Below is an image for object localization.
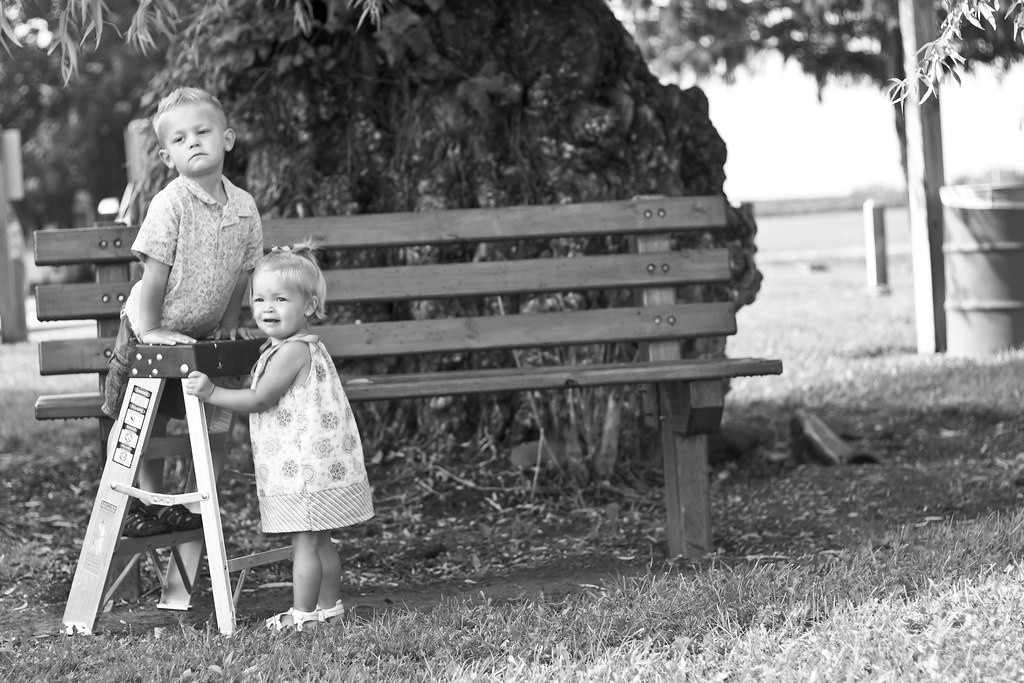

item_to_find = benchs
[35,195,784,607]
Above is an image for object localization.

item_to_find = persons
[185,243,377,631]
[97,86,265,537]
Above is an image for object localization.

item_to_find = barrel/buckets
[940,180,1022,356]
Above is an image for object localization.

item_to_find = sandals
[122,502,171,536]
[317,600,350,626]
[266,606,320,634]
[154,504,204,530]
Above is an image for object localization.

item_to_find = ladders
[60,346,241,639]
